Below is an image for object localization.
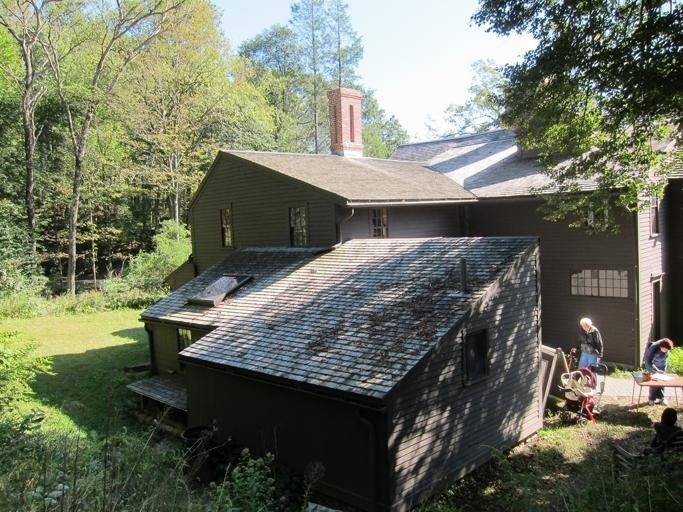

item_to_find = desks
[630,372,683,417]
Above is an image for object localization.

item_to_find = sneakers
[649,398,668,406]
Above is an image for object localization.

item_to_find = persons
[644,336,675,406]
[571,317,604,389]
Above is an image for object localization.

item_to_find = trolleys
[561,360,610,428]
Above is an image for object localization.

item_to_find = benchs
[609,441,683,486]
